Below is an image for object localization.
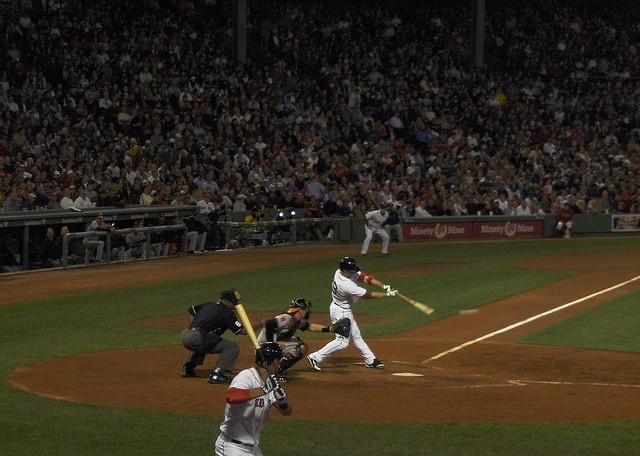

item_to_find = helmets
[286,298,312,313]
[340,256,361,272]
[256,342,283,360]
[221,289,240,305]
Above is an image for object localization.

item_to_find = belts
[230,439,255,446]
[334,301,351,309]
[189,327,210,332]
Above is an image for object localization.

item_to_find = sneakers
[181,366,196,376]
[278,375,288,383]
[307,355,322,371]
[207,372,232,383]
[366,358,385,369]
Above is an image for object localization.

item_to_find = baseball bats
[234,303,272,375]
[396,292,434,315]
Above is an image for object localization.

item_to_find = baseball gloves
[331,318,350,338]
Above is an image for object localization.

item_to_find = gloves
[386,289,398,297]
[383,285,391,290]
[273,386,287,405]
[261,374,279,394]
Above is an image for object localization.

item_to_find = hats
[381,202,387,208]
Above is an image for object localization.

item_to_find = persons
[168,216,186,255]
[180,289,266,384]
[384,205,403,243]
[576,200,640,216]
[360,201,390,256]
[57,226,72,256]
[555,0,640,201]
[125,221,150,258]
[154,213,171,257]
[271,209,287,245]
[240,208,268,248]
[306,256,398,370]
[215,342,293,456]
[41,228,60,262]
[556,202,575,238]
[84,212,116,263]
[186,214,208,255]
[254,297,350,383]
[303,195,323,238]
[286,207,298,241]
[323,192,350,240]
